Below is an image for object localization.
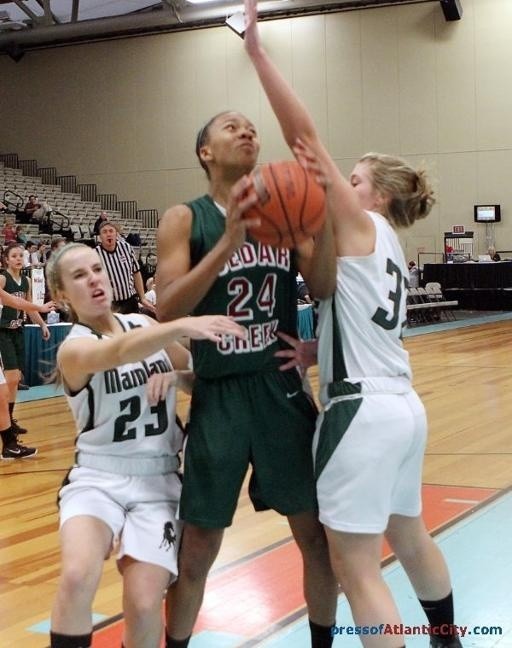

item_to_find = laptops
[479,255,493,262]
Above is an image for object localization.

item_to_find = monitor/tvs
[474,204,500,223]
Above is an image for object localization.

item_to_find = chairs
[0,162,157,255]
[404,284,458,326]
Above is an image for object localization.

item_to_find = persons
[46,240,247,648]
[231,0,463,648]
[486,246,501,262]
[155,111,340,647]
[296,280,312,304]
[408,260,419,288]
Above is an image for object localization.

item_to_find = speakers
[440,0,463,21]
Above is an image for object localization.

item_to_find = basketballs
[242,159,327,248]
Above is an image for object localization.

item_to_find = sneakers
[2,446,38,459]
[12,420,27,434]
